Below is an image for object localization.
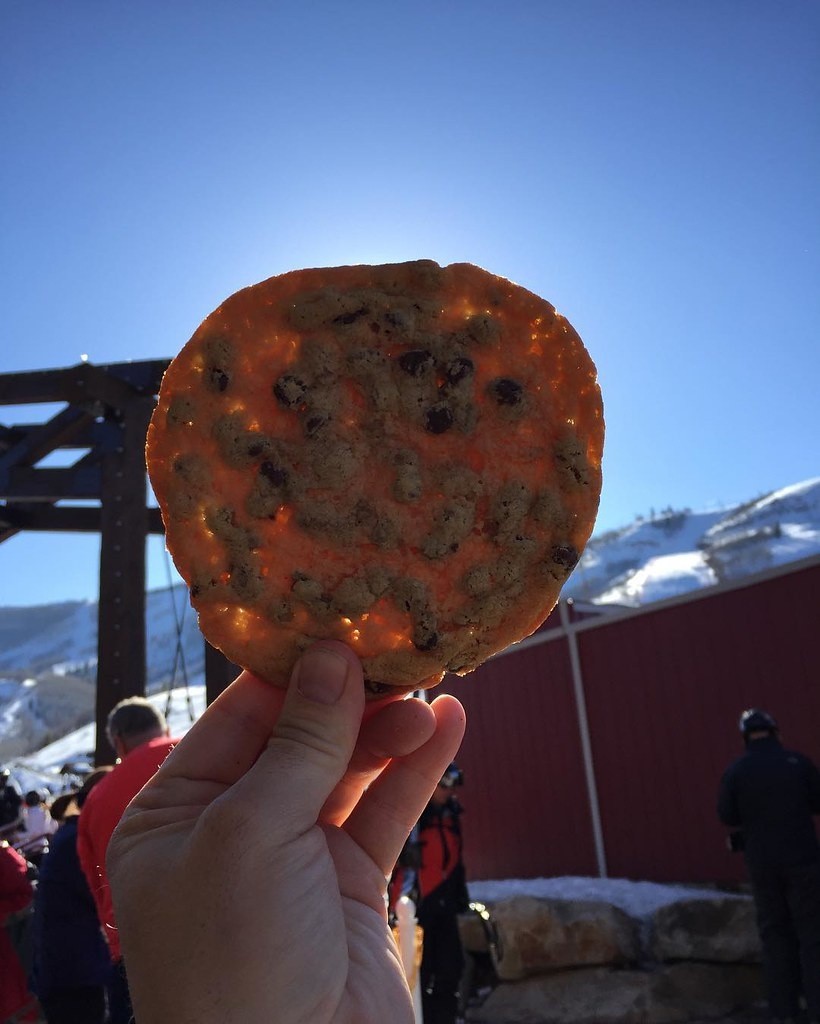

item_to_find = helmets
[738,708,777,739]
[439,764,466,790]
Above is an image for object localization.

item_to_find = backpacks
[455,903,504,1005]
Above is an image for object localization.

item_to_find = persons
[719,709,820,1016]
[76,697,191,973]
[102,655,467,1024]
[386,755,475,1024]
[0,697,139,1024]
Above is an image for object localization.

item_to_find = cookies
[145,259,607,699]
